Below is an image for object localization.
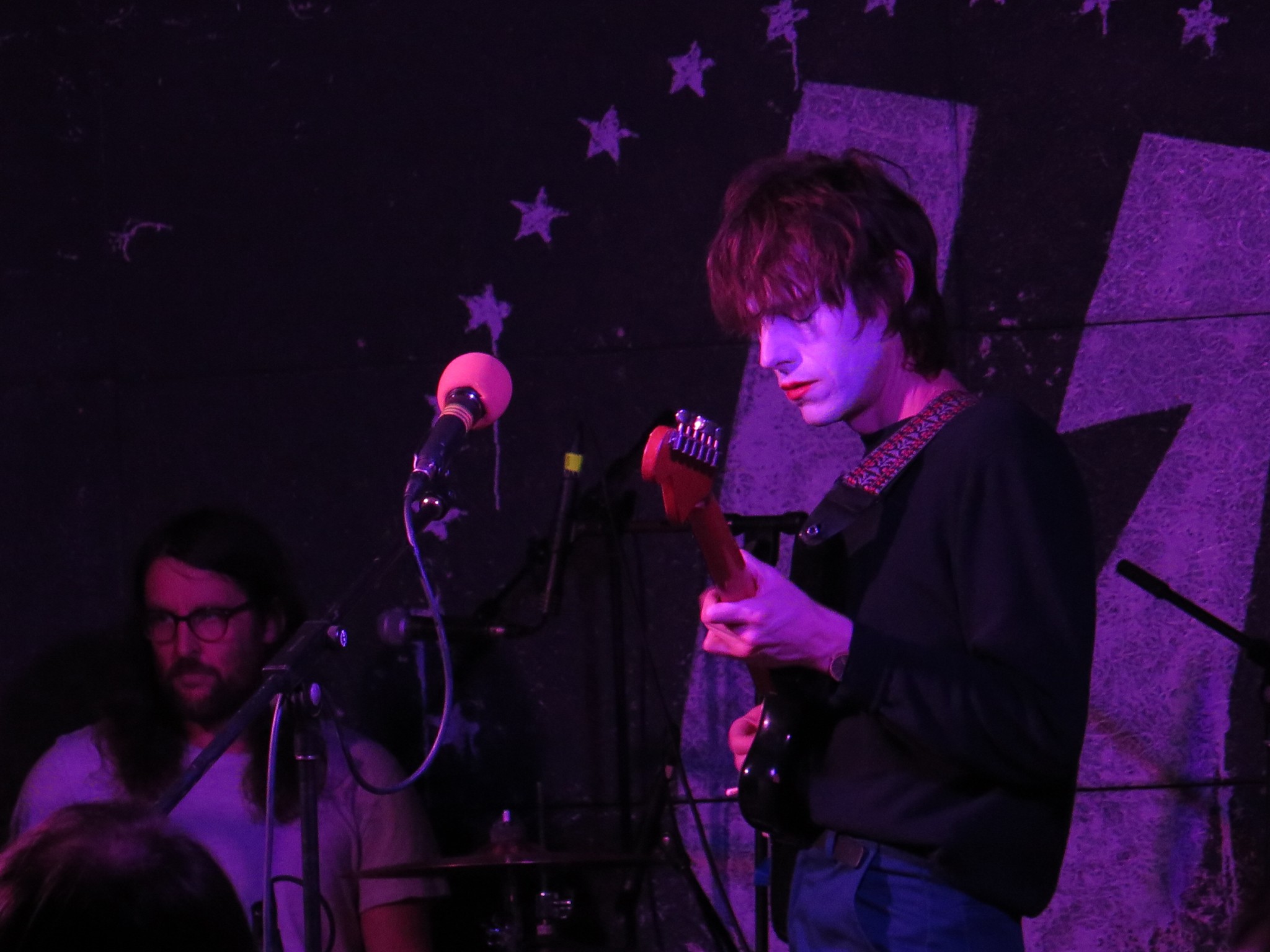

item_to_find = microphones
[374,609,510,645]
[405,352,514,497]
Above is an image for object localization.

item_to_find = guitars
[640,410,833,944]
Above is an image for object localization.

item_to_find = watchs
[825,651,851,683]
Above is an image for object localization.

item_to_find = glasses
[134,599,256,642]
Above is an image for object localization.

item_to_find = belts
[784,824,950,884]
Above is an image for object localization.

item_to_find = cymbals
[357,843,664,879]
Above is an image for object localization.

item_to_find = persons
[696,149,1095,952]
[0,509,452,952]
[0,799,265,952]
